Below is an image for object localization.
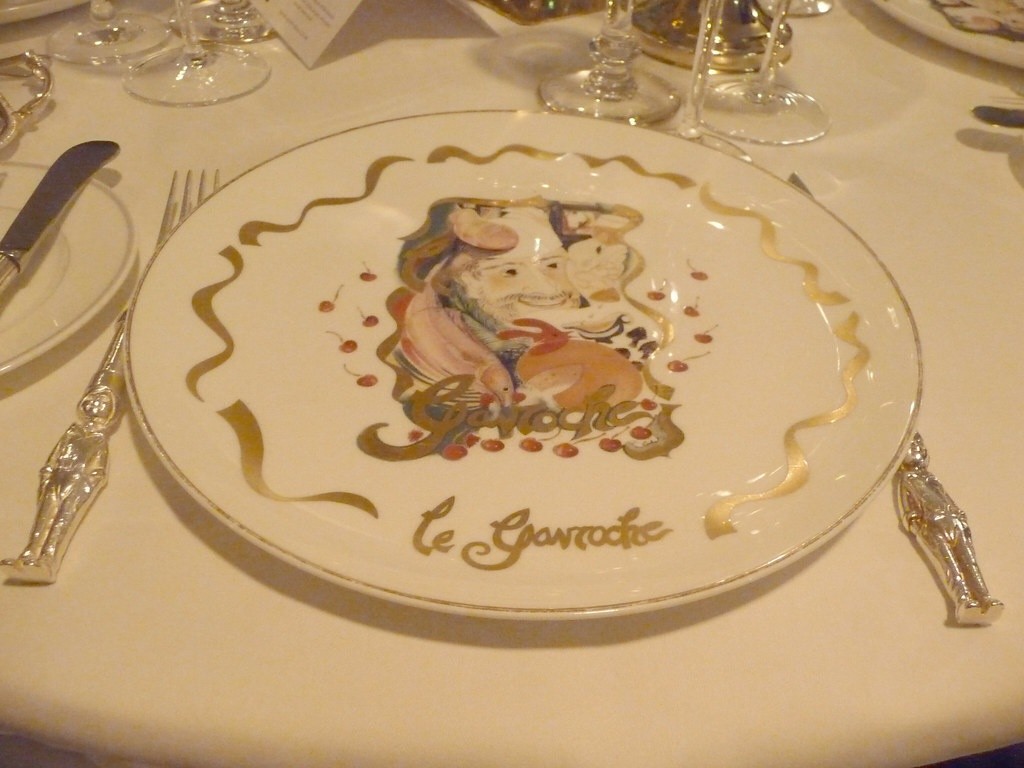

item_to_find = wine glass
[44,1,173,67]
[123,0,273,106]
[169,0,274,44]
[658,0,752,162]
[537,0,682,124]
[696,0,832,145]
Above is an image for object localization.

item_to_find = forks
[1,169,223,583]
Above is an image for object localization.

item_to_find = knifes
[788,173,1002,626]
[0,141,120,292]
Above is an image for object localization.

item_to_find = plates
[0,164,140,376]
[122,110,924,620]
[871,0,1024,70]
